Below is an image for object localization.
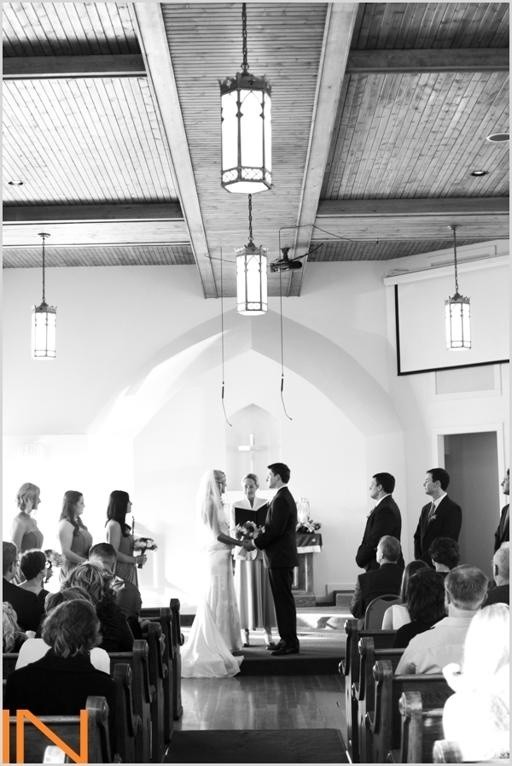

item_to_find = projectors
[271,250,304,274]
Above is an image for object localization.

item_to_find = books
[234,501,269,529]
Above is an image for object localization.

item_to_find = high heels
[264,632,274,646]
[243,632,249,646]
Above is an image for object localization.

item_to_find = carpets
[163,726,349,763]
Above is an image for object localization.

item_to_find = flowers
[133,536,159,569]
[233,521,258,560]
[44,549,64,583]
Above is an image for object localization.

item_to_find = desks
[292,531,322,595]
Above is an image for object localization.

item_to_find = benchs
[337,615,458,762]
[4,595,199,762]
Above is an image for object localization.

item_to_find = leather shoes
[266,639,283,650]
[272,642,299,655]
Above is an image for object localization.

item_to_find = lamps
[234,192,270,316]
[31,234,57,360]
[444,223,475,350]
[217,2,274,195]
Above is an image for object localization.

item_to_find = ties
[427,504,435,521]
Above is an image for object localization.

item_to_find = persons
[4,539,166,764]
[493,469,509,551]
[13,484,44,559]
[105,491,146,589]
[201,468,247,657]
[414,466,463,563]
[231,472,276,651]
[343,532,511,762]
[242,462,300,655]
[355,472,405,575]
[58,491,93,574]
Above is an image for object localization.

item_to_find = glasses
[44,562,50,569]
